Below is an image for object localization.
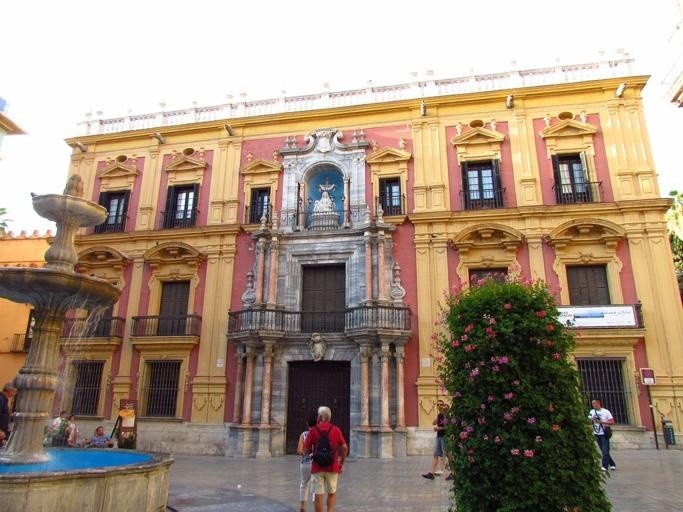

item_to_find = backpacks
[312,423,337,469]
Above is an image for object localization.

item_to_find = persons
[296,413,315,512]
[421,399,455,480]
[433,404,452,476]
[52,410,66,429]
[312,191,336,214]
[587,399,616,470]
[303,406,348,512]
[66,415,79,446]
[89,425,113,447]
[0,382,16,447]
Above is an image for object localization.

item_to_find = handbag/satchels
[603,427,613,440]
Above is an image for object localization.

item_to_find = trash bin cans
[662,419,675,445]
[51,434,65,447]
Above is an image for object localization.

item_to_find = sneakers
[601,463,617,472]
[420,469,456,481]
